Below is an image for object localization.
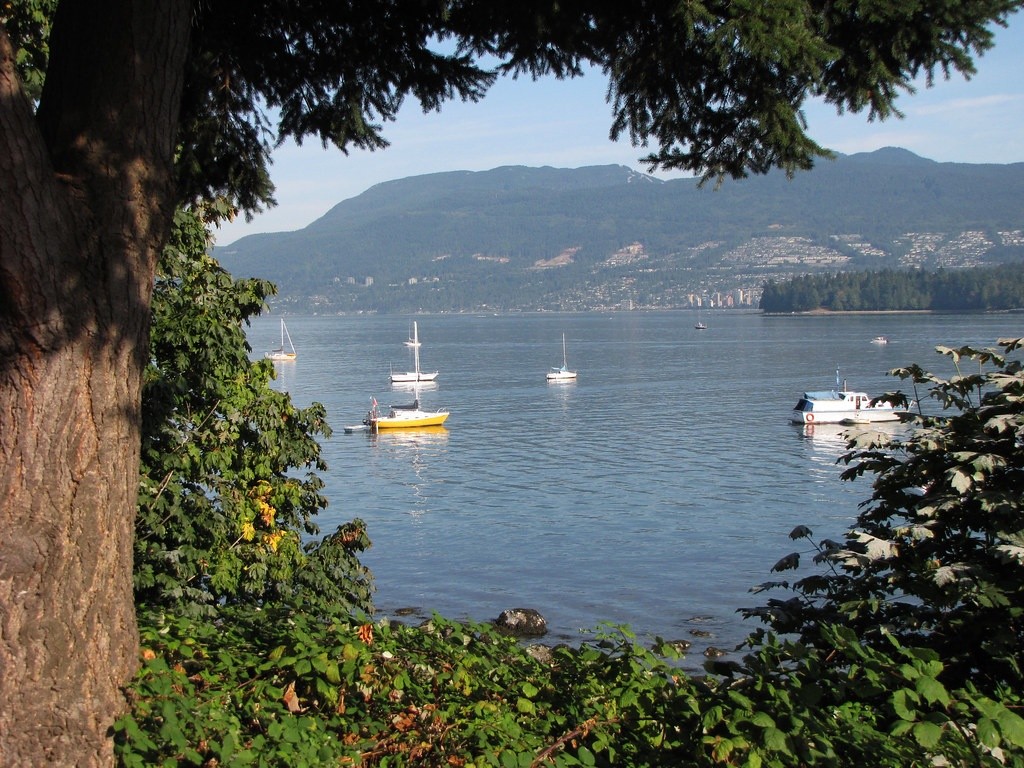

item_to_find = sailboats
[263,316,299,361]
[401,318,422,347]
[544,332,579,380]
[387,321,440,382]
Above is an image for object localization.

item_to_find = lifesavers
[806,424,814,430]
[807,414,814,422]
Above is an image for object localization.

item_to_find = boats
[343,423,370,433]
[870,336,889,345]
[785,366,917,426]
[694,321,708,330]
[362,372,449,429]
